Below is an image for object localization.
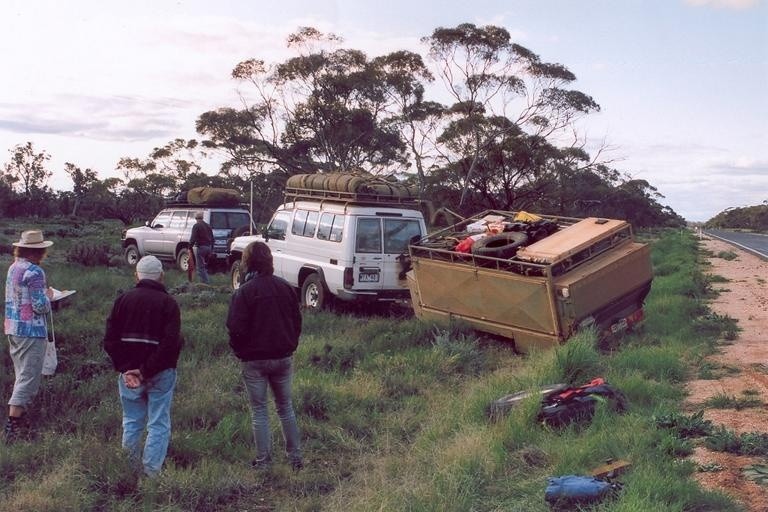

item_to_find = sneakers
[250,455,274,467]
[290,457,304,470]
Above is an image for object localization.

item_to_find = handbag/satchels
[40,340,59,376]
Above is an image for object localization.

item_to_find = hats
[11,229,55,250]
[135,255,164,282]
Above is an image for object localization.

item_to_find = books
[49,286,76,302]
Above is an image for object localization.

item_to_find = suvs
[226,187,431,315]
[118,196,262,276]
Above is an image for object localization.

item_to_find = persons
[3,229,54,434]
[104,255,181,476]
[188,213,214,284]
[226,241,302,465]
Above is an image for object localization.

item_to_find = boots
[4,416,39,442]
